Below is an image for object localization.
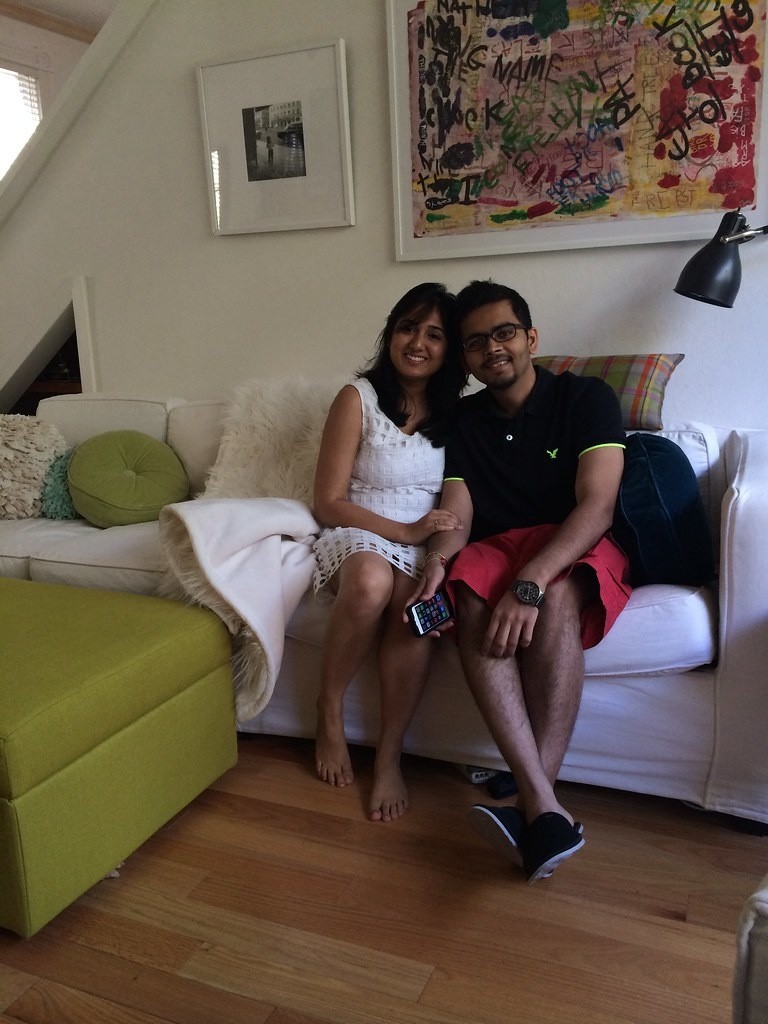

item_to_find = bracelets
[423,550,448,568]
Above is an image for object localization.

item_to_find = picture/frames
[386,0,768,263]
[195,38,356,238]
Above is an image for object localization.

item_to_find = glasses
[462,323,525,352]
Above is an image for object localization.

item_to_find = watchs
[509,579,545,609]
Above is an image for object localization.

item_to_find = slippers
[470,804,524,867]
[522,812,584,888]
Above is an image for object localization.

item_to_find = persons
[265,135,274,162]
[401,277,633,883]
[290,282,460,821]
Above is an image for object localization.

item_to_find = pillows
[43,445,78,521]
[0,413,69,521]
[67,427,190,529]
[530,353,686,431]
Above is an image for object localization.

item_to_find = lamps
[674,201,768,309]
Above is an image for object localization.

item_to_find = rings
[435,519,438,525]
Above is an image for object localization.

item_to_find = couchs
[0,392,767,827]
[0,576,242,938]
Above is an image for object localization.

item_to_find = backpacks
[611,432,713,587]
